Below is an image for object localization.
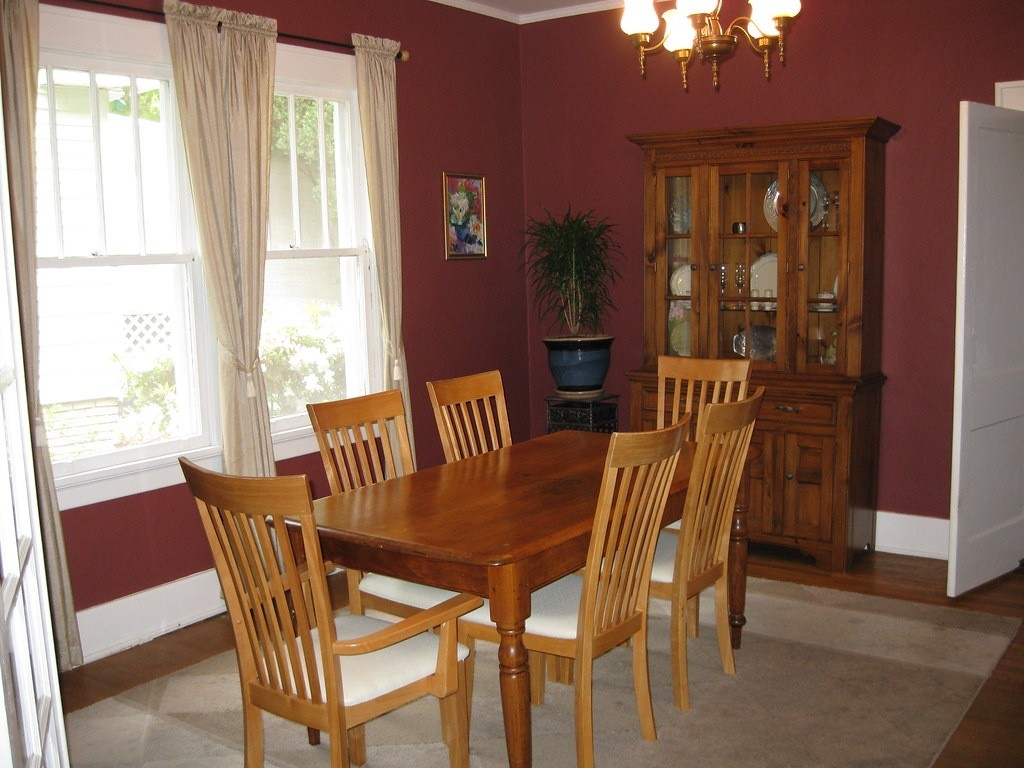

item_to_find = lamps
[620,1,804,92]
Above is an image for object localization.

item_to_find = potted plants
[521,206,623,397]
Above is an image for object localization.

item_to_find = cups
[732,222,746,233]
[749,288,759,310]
[816,290,834,311]
[764,289,774,310]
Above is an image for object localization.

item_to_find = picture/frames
[442,171,490,261]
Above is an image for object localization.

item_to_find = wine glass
[732,262,746,310]
[718,262,729,310]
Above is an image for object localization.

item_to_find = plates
[672,321,691,357]
[750,252,778,309]
[733,326,776,360]
[670,265,690,311]
[763,172,828,234]
[669,192,690,233]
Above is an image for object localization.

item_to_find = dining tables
[262,417,762,768]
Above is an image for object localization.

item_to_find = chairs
[453,408,695,768]
[175,452,474,766]
[305,387,482,741]
[636,354,754,641]
[428,368,515,461]
[581,381,768,713]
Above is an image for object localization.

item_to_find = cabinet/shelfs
[629,113,901,577]
